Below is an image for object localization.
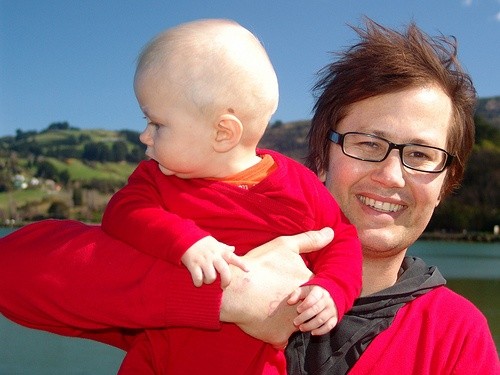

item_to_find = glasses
[328,128,458,174]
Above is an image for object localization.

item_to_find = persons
[101,17,363,375]
[0,13,500,375]
